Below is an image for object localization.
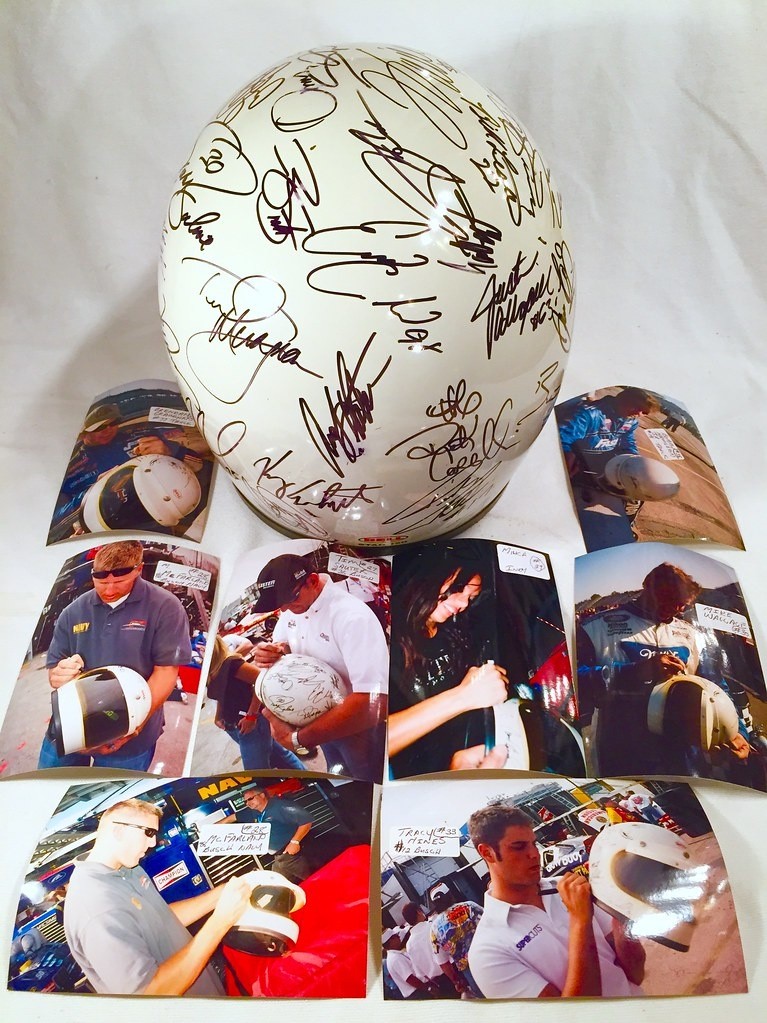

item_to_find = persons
[574,561,751,778]
[37,538,195,772]
[387,547,513,783]
[576,603,620,626]
[50,402,212,543]
[59,798,252,999]
[212,786,318,887]
[203,554,390,784]
[382,789,664,1000]
[556,386,687,500]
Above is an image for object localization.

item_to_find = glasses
[91,418,121,432]
[111,821,159,839]
[244,792,262,803]
[286,576,307,605]
[91,560,143,579]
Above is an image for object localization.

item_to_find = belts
[223,724,240,732]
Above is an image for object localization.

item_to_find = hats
[382,925,402,944]
[430,883,450,901]
[251,553,316,615]
[81,403,125,432]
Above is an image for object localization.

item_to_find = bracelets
[247,712,260,717]
[245,715,258,721]
[427,986,431,991]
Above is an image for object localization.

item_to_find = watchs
[291,731,310,755]
[290,840,300,845]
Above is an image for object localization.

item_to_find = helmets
[646,674,738,751]
[255,653,347,729]
[154,44,576,549]
[465,697,586,777]
[604,453,680,502]
[584,823,712,952]
[49,664,152,759]
[219,868,305,957]
[78,453,201,533]
